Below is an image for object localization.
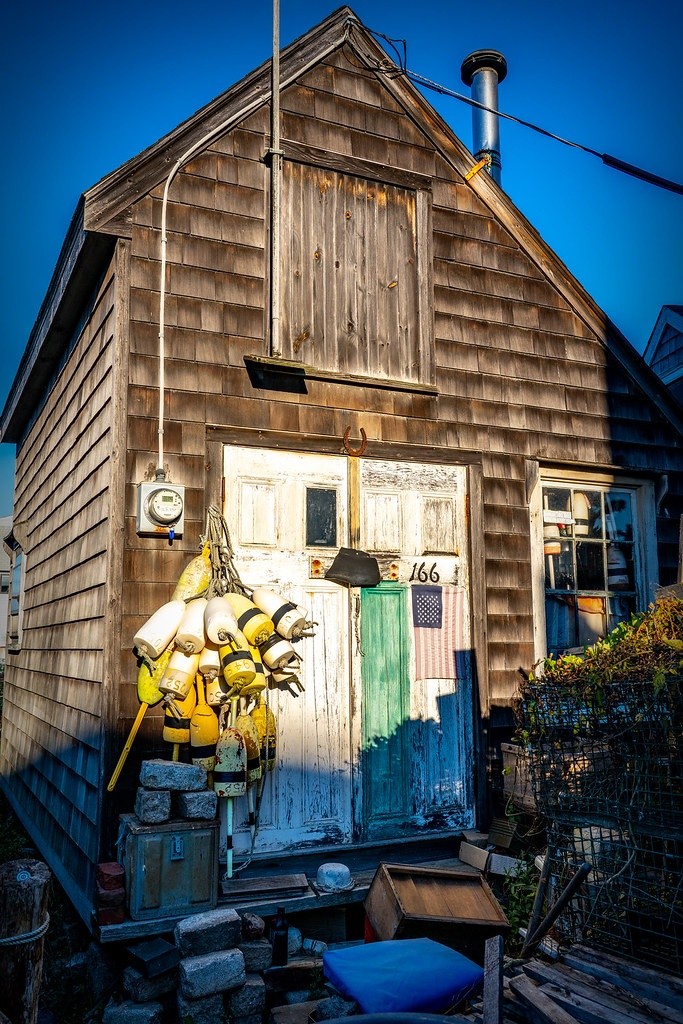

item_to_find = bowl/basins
[313,863,354,893]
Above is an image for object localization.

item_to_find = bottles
[269,906,289,966]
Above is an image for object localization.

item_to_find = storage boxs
[459,831,527,877]
[363,861,511,952]
